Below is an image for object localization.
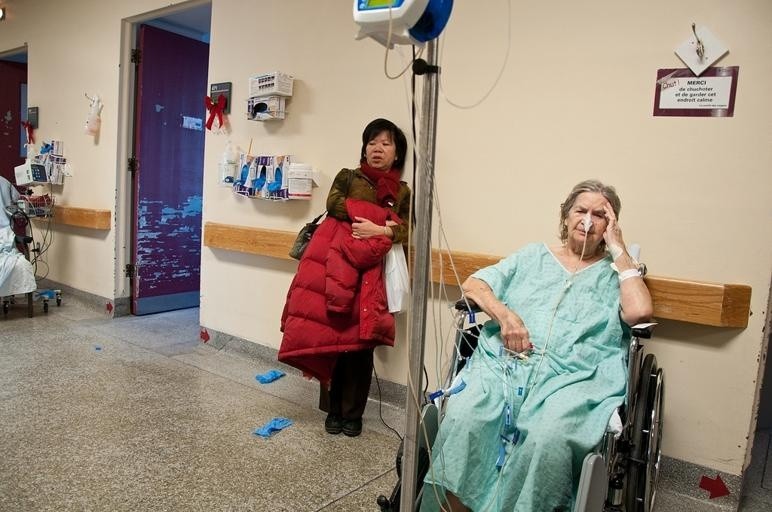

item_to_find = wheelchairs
[376,297,665,512]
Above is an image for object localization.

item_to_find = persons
[318,118,417,436]
[0,175,37,299]
[443,179,654,512]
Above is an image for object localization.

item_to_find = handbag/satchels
[288,222,320,260]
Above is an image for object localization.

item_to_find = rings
[357,232,361,235]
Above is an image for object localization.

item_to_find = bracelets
[383,225,388,236]
[619,268,642,283]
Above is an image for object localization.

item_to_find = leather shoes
[325,412,362,437]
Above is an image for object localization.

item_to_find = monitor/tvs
[354,0,453,44]
